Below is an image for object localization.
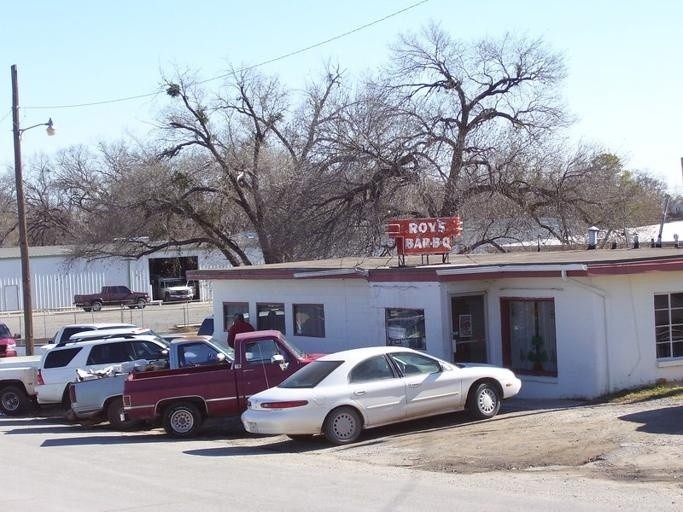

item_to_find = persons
[227,313,256,349]
[300,308,324,336]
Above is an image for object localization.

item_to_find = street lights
[12,115,57,357]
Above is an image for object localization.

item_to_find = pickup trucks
[71,285,149,313]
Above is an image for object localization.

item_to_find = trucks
[153,274,197,303]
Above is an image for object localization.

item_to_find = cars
[238,344,522,449]
